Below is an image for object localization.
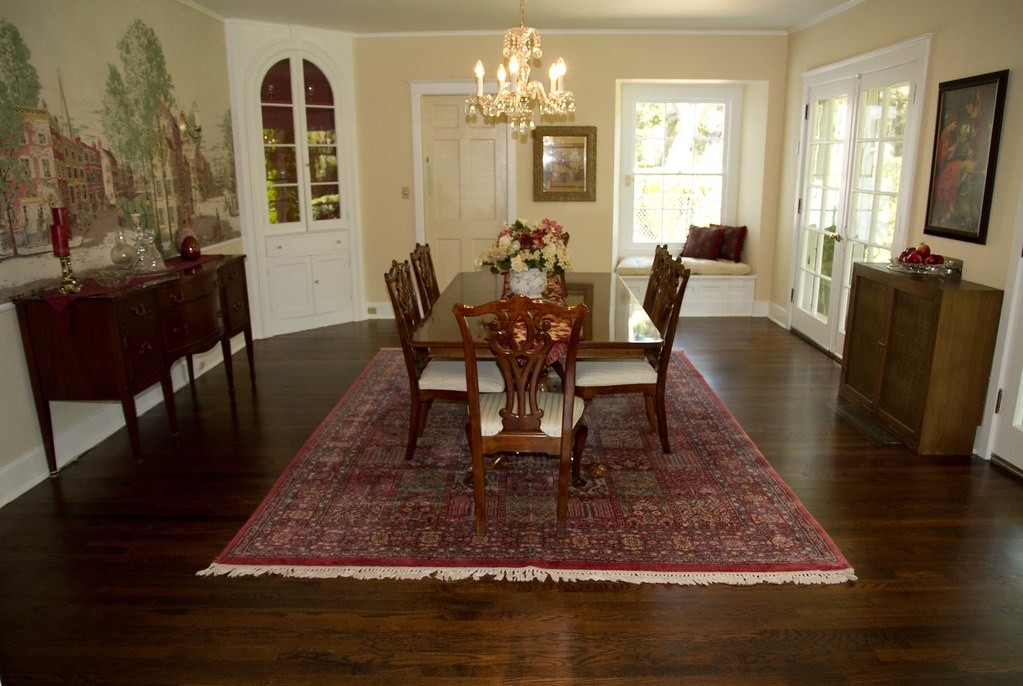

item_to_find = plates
[886,260,955,280]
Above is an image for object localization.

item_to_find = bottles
[111,225,166,275]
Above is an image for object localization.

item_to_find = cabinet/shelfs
[9,254,255,478]
[837,261,1004,457]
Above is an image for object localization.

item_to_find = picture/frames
[531,125,597,202]
[922,69,1009,245]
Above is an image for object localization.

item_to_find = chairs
[384,259,505,460]
[642,244,672,319]
[410,242,441,317]
[451,294,590,539]
[575,256,691,453]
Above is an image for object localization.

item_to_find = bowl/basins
[89,263,141,287]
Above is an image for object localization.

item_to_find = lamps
[464,0,576,132]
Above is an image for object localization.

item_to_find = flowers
[472,216,573,278]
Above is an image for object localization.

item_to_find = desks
[407,272,663,359]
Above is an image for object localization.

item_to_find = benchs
[616,255,757,318]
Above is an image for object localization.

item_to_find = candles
[53,206,72,238]
[49,224,70,255]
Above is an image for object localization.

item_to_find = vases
[508,268,549,300]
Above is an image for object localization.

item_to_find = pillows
[679,225,726,262]
[710,223,748,263]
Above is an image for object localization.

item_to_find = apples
[899,243,944,264]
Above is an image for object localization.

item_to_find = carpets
[196,341,859,586]
